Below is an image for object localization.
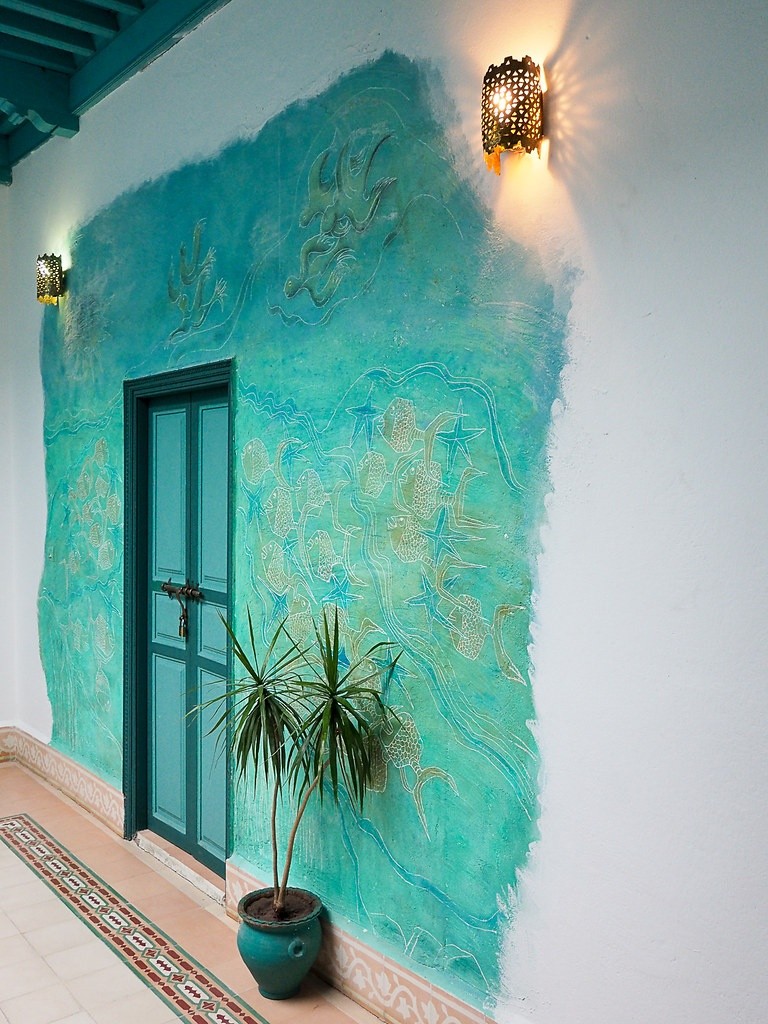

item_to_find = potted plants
[184,601,415,1001]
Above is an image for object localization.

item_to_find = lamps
[36,252,66,306]
[481,54,544,176]
